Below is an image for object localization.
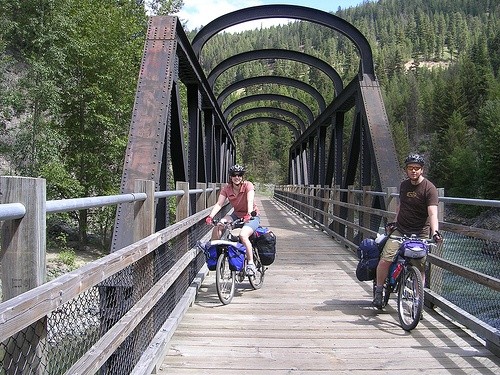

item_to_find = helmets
[405,154,424,166]
[230,165,245,174]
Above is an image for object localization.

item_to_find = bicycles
[215,218,268,305]
[370,225,443,331]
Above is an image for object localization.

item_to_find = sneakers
[246,264,256,276]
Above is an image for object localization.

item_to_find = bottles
[392,262,404,280]
[390,263,397,284]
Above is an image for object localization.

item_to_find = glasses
[407,166,421,171]
[231,174,242,177]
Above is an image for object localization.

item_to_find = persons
[372,154,444,319]
[205,165,260,275]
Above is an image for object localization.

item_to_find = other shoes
[372,297,384,307]
[411,307,423,319]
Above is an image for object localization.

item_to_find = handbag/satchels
[355,237,380,281]
[204,241,222,270]
[229,243,247,270]
[400,240,427,260]
[255,229,276,265]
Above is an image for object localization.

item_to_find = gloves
[244,213,251,223]
[206,216,213,224]
